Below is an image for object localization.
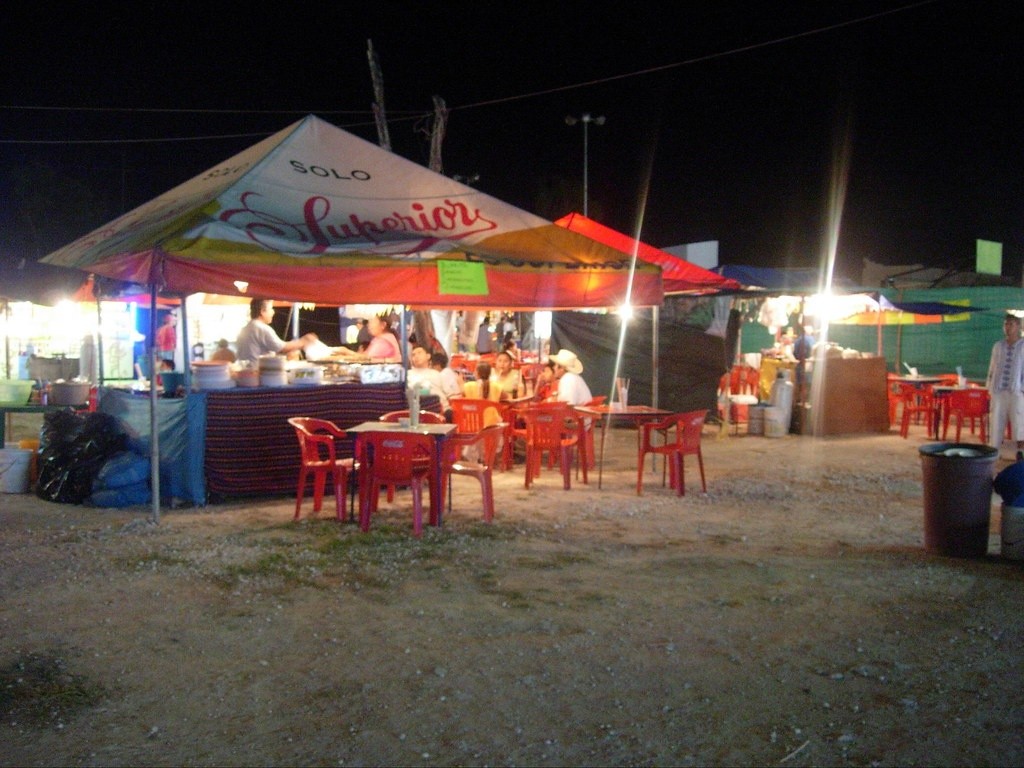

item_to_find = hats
[355,319,368,325]
[163,314,178,321]
[549,348,584,374]
[214,338,228,347]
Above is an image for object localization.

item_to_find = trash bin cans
[918,441,1001,560]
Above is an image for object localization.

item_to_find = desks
[572,403,670,490]
[342,420,454,533]
[935,384,964,440]
[884,373,942,430]
[0,403,86,453]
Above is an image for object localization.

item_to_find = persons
[404,311,593,459]
[211,338,236,363]
[792,326,812,409]
[332,314,401,364]
[235,296,318,360]
[134,313,178,385]
[761,325,792,359]
[986,315,1024,461]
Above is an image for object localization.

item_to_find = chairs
[285,354,713,533]
[720,344,1011,446]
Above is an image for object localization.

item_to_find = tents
[38,115,662,517]
[518,213,1024,438]
